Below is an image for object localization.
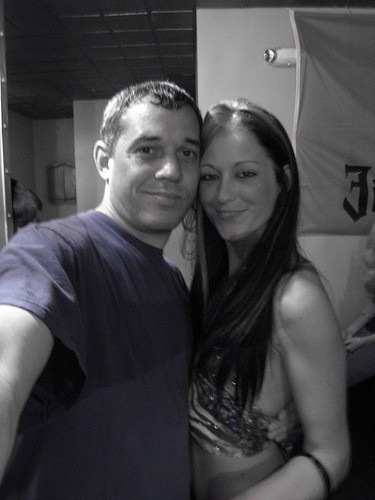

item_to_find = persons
[340,222,375,354]
[0,81,203,499]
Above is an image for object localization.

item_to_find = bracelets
[183,96,352,500]
[361,312,371,320]
